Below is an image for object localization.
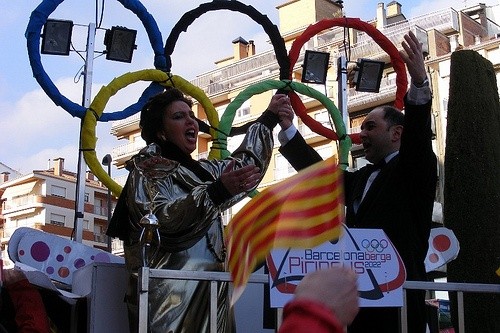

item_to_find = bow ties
[366,159,386,171]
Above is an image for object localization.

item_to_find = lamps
[102,25,138,64]
[353,58,384,93]
[301,50,330,84]
[41,18,74,57]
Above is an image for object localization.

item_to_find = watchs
[411,75,429,89]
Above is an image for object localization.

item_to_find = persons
[104,86,290,333]
[277,30,438,333]
[0,270,50,333]
[278,266,360,333]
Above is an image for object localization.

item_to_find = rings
[409,52,415,58]
[240,181,247,187]
